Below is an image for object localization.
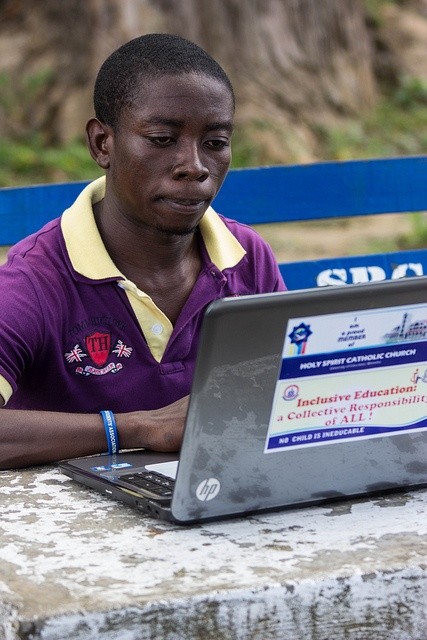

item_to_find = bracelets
[100,408,119,456]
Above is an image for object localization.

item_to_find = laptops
[55,275,427,526]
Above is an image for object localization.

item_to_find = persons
[0,34,289,472]
